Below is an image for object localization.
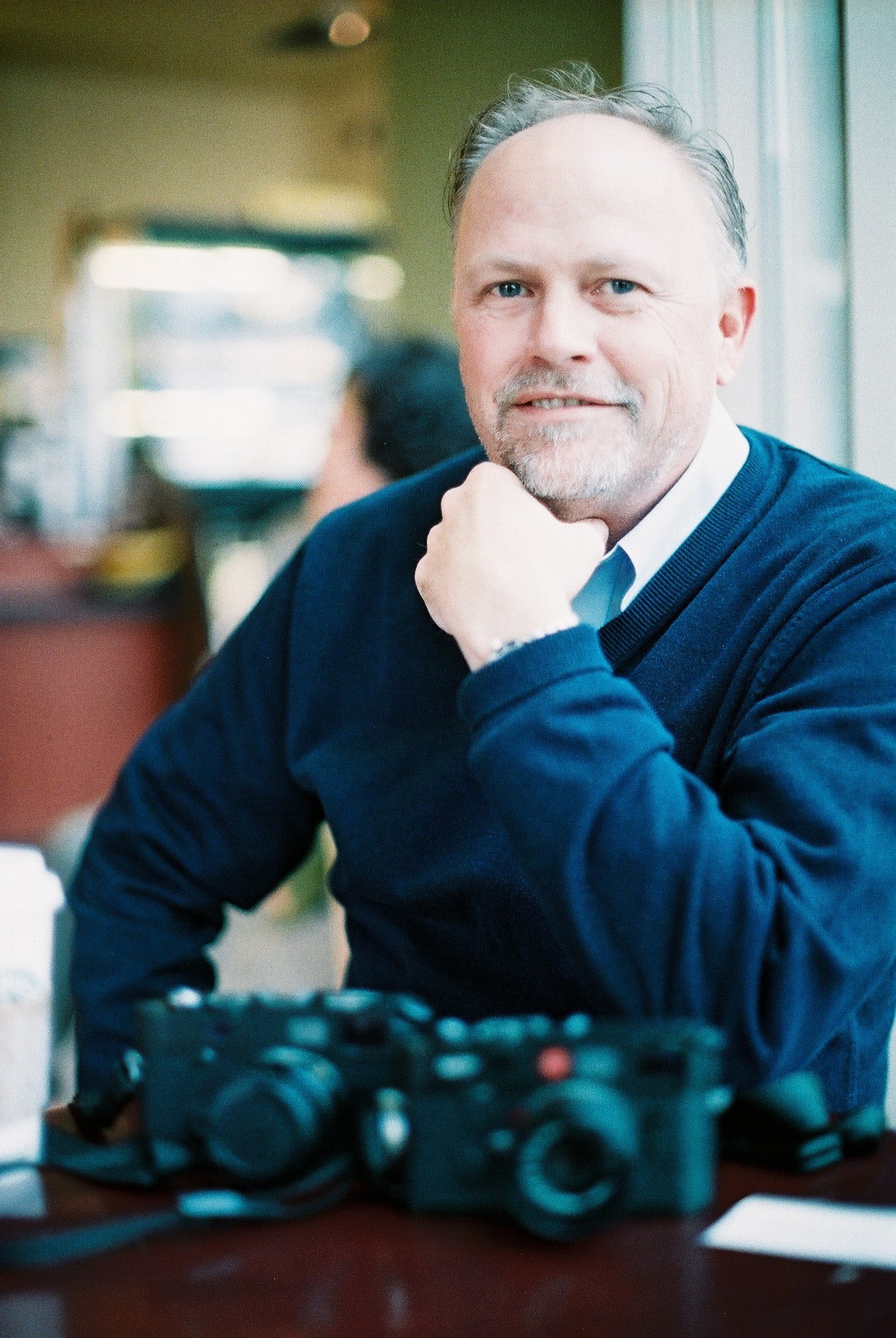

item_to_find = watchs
[490,641,530,659]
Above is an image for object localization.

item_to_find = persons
[65,59,896,1118]
[310,325,481,528]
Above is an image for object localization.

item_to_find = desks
[0,1097,896,1338]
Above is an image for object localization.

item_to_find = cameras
[137,987,433,1189]
[405,1014,742,1244]
